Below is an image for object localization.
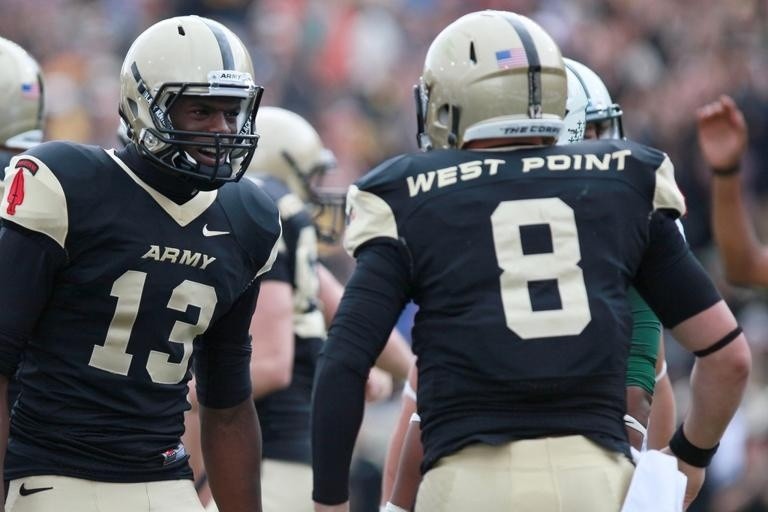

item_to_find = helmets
[117,14,258,156]
[412,8,626,152]
[233,104,336,204]
[0,36,48,151]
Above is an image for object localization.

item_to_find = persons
[1,2,768,512]
